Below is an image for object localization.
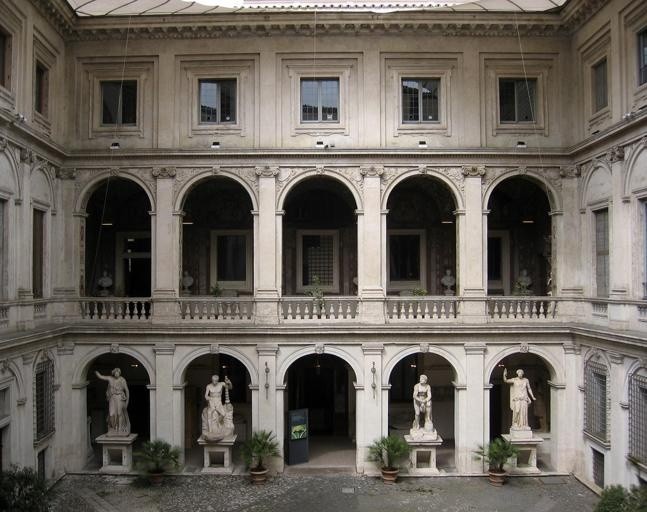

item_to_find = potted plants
[472,437,521,486]
[136,441,180,486]
[239,431,283,483]
[366,434,412,484]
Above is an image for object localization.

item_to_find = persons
[503,366,535,431]
[412,374,434,432]
[204,375,232,432]
[94,368,130,433]
